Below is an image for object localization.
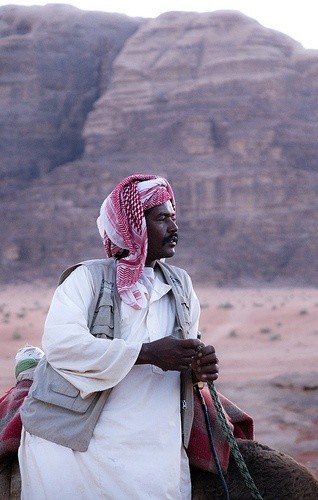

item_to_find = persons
[17,174,218,500]
